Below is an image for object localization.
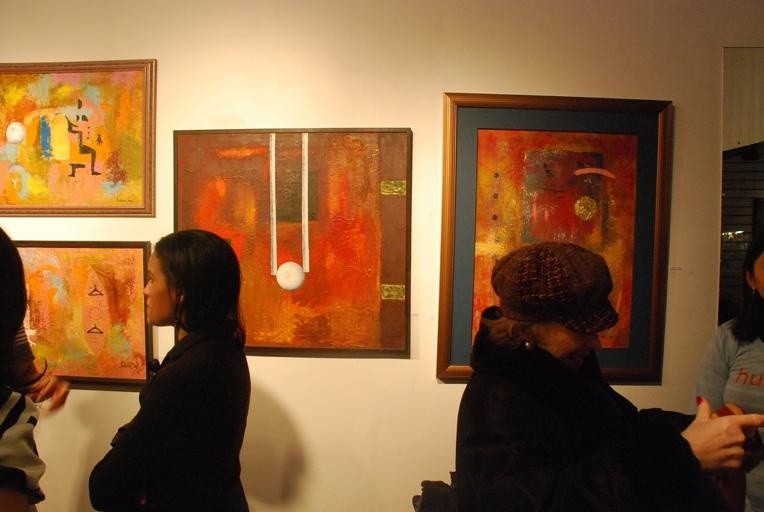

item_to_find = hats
[489,238,621,336]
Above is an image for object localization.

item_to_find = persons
[89,229,250,509]
[0,228,47,511]
[454,240,764,511]
[14,326,71,414]
[694,238,764,511]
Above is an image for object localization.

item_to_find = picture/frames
[170,128,414,362]
[9,237,154,390]
[0,62,157,218]
[436,88,676,386]
